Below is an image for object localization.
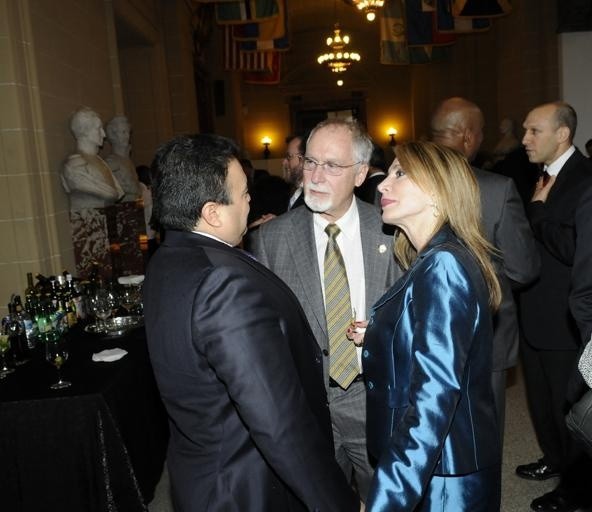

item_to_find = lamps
[260,135,271,159]
[349,0,385,21]
[388,127,398,146]
[317,21,360,87]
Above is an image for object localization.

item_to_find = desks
[0,267,167,509]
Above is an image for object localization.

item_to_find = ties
[323,224,360,390]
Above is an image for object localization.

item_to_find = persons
[562,179,591,453]
[143,132,366,512]
[104,116,140,201]
[429,96,542,455]
[135,117,592,253]
[60,106,125,208]
[346,143,503,512]
[515,101,591,511]
[251,118,417,500]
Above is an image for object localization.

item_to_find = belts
[328,375,365,385]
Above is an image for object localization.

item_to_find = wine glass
[86,286,143,337]
[46,330,72,389]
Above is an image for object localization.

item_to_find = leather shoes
[518,459,560,480]
[531,488,579,511]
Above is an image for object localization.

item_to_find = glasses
[285,151,301,161]
[300,157,364,174]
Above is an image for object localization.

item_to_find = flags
[215,0,291,87]
[379,0,511,67]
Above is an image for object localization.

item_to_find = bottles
[40,271,86,335]
[0,272,40,382]
